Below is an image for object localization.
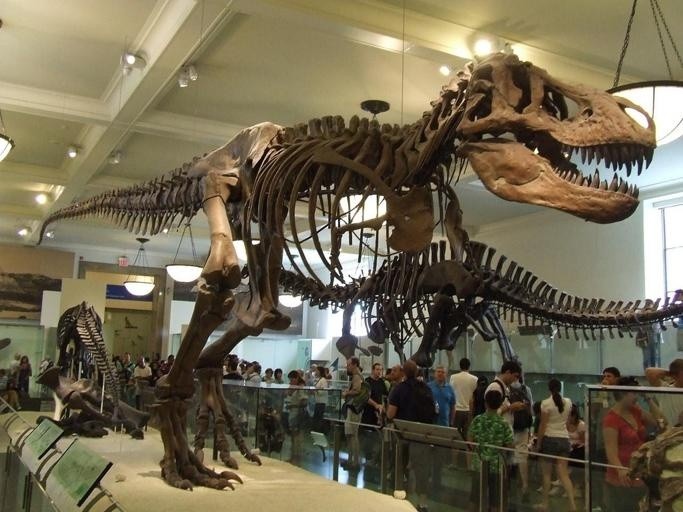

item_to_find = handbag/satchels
[348,373,372,414]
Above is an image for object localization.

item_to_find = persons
[668,288,683,353]
[223,344,683,510]
[112,353,175,389]
[634,299,664,372]
[7,353,50,399]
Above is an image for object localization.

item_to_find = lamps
[164,217,204,284]
[122,237,157,297]
[126,54,147,70]
[112,150,122,164]
[66,145,80,158]
[0,132,15,163]
[178,65,198,89]
[277,254,303,309]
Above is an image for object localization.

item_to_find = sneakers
[537,477,582,499]
[521,487,531,504]
[533,504,550,511]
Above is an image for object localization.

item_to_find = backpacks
[473,380,505,418]
[507,383,533,431]
